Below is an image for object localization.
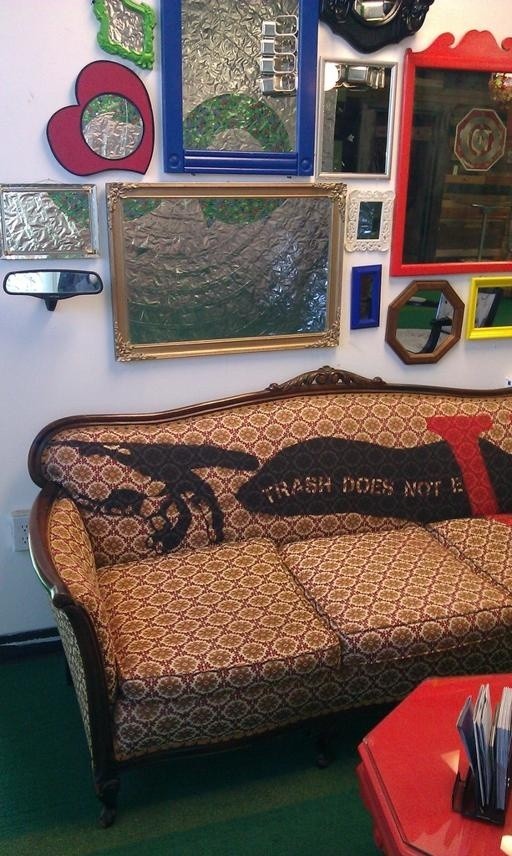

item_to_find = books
[456,682,512,810]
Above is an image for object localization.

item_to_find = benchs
[23,366,512,832]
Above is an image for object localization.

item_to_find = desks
[354,672,512,855]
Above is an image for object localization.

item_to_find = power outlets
[12,510,30,554]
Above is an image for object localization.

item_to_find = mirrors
[389,24,512,277]
[4,268,104,314]
[385,275,512,366]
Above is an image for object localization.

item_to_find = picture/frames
[105,180,349,364]
[157,0,320,187]
[350,263,383,330]
[347,188,396,254]
[0,181,100,261]
[321,0,436,55]
[315,56,399,181]
[45,57,155,177]
[88,0,157,74]
